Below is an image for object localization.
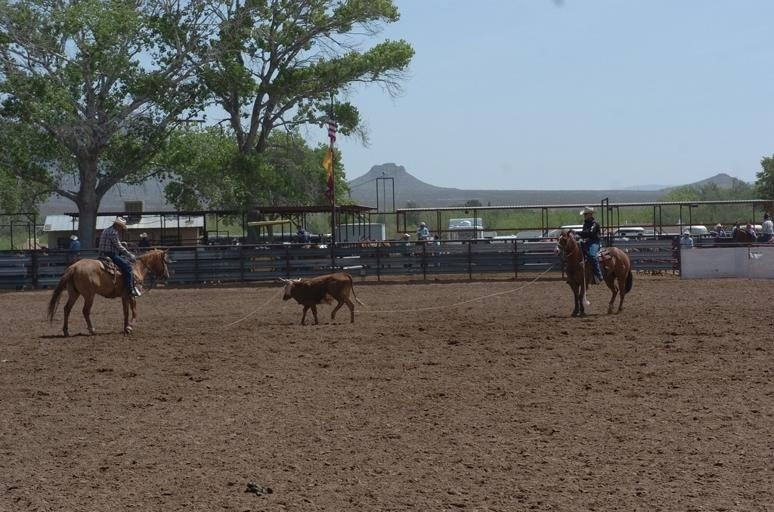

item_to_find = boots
[123,272,137,297]
[593,257,603,280]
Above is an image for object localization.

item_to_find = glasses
[684,234,689,235]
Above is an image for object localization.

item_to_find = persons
[17,235,81,269]
[538,212,774,270]
[99,217,142,297]
[296,225,306,240]
[569,207,605,285]
[140,232,151,246]
[399,222,439,268]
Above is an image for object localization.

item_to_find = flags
[322,148,335,203]
[327,94,337,142]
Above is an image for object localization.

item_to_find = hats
[714,224,722,230]
[112,217,128,229]
[580,207,594,215]
[683,229,690,235]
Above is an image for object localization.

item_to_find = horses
[733,228,773,242]
[46,246,172,336]
[671,236,680,276]
[554,227,632,317]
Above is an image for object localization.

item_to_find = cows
[277,271,365,325]
